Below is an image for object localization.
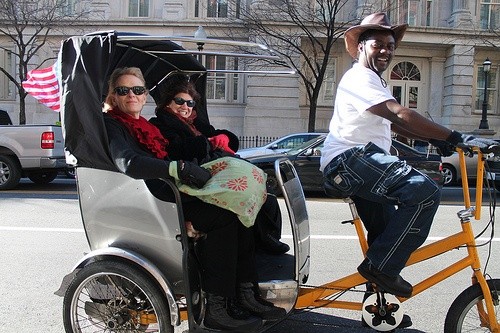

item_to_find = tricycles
[51,28,500,333]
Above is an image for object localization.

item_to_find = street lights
[478,56,492,129]
[194,25,207,65]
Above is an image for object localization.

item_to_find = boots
[203,293,263,333]
[235,280,286,318]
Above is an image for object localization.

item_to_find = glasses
[172,97,196,107]
[114,86,147,96]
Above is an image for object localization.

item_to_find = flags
[22,65,60,112]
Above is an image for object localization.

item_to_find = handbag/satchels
[177,156,267,227]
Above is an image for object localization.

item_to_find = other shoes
[263,234,290,254]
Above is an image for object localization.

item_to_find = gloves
[176,160,211,188]
[445,130,475,157]
[208,134,235,154]
[429,138,455,157]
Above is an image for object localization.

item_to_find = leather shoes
[362,306,412,328]
[357,257,413,298]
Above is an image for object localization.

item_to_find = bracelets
[446,130,462,145]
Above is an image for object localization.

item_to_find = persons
[318,12,500,329]
[102,67,290,333]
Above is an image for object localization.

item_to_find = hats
[344,12,408,59]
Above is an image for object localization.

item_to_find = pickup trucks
[0,109,76,191]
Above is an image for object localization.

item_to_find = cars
[438,133,500,194]
[235,131,444,198]
